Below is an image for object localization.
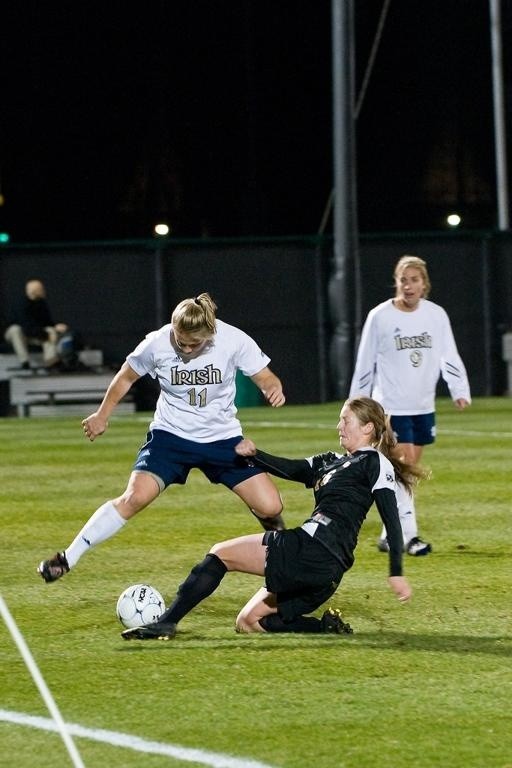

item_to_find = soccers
[116,584,165,629]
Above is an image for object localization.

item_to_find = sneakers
[36,550,72,584]
[405,536,432,557]
[377,535,391,552]
[119,618,177,642]
[320,607,355,637]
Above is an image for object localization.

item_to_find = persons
[4,277,70,369]
[36,289,288,584]
[119,395,412,642]
[344,251,475,560]
